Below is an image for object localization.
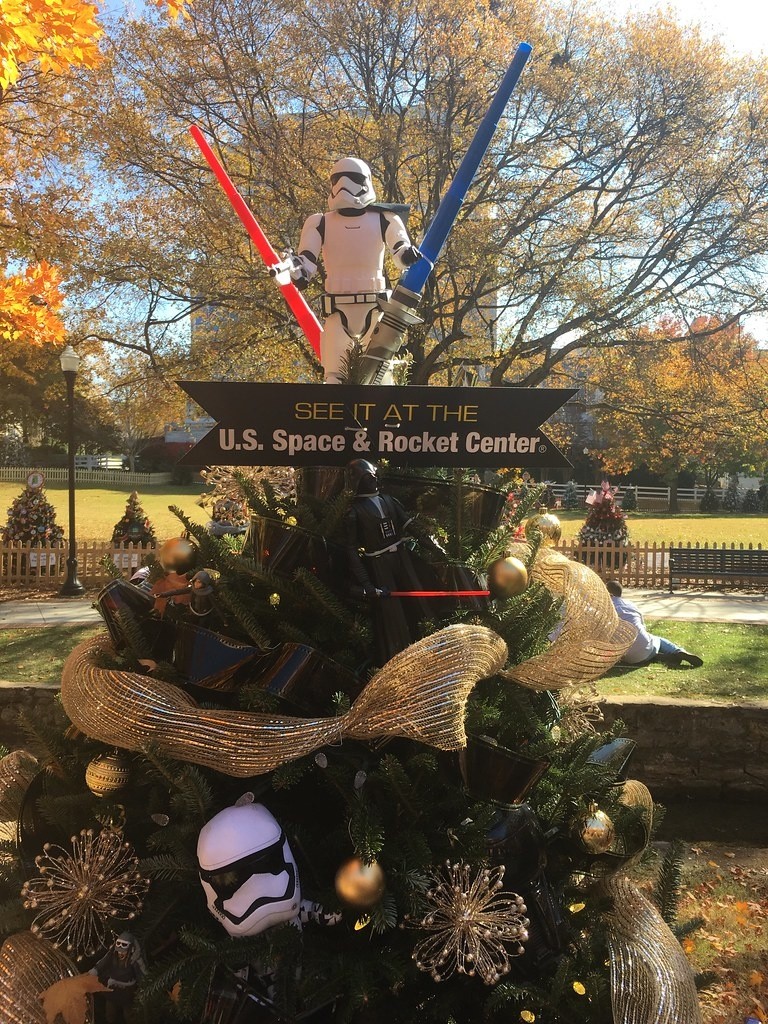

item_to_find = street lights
[56,343,87,595]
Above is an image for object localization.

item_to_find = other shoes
[664,656,682,664]
[678,651,703,666]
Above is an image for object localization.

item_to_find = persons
[344,458,453,650]
[195,799,303,939]
[89,932,148,1024]
[208,498,246,539]
[154,571,229,628]
[291,155,424,387]
[547,581,703,668]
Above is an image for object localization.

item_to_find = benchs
[669,547,768,595]
